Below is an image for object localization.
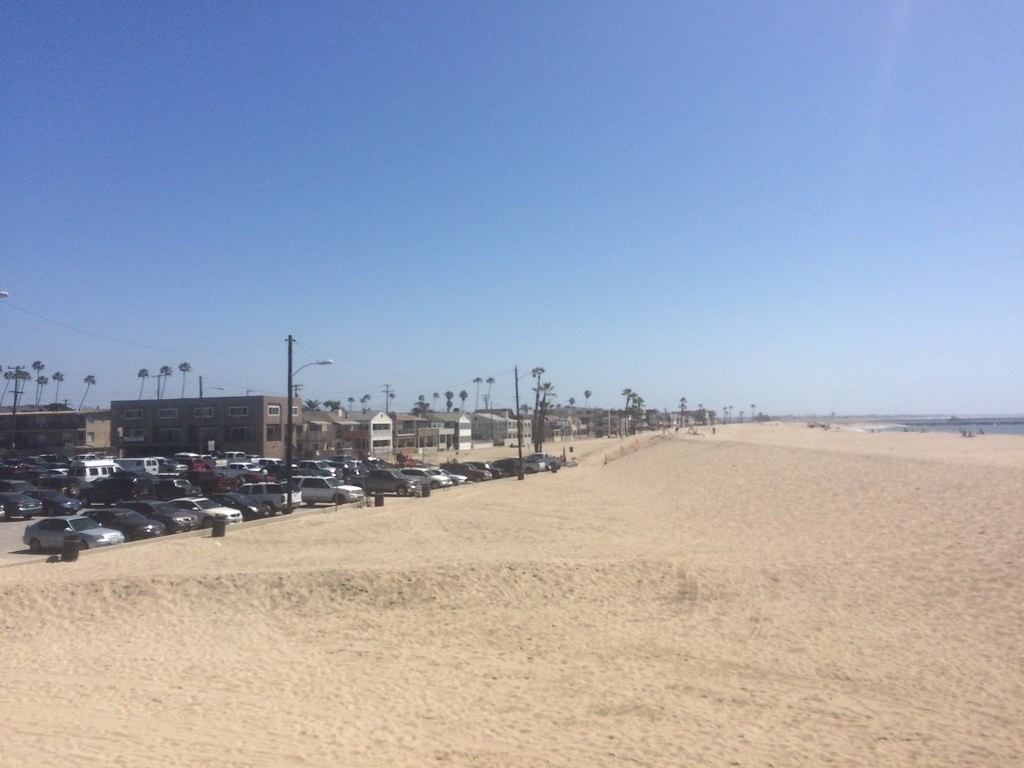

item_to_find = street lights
[286,359,334,512]
[197,386,223,455]
[515,370,542,480]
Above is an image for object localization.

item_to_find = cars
[1,449,564,554]
